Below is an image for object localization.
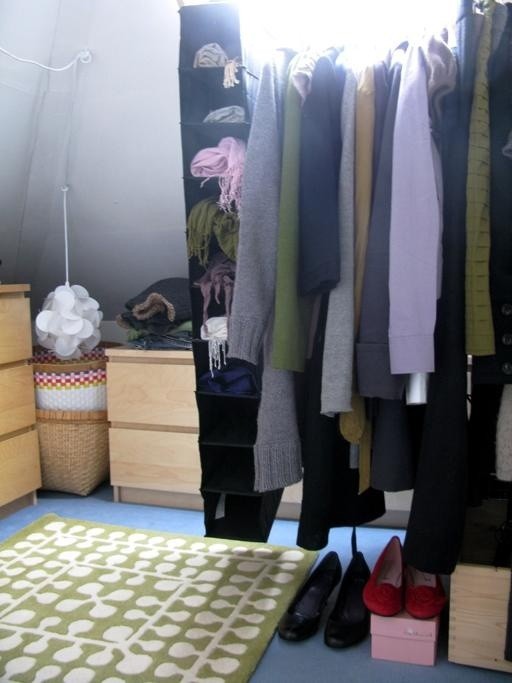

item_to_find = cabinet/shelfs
[101,347,415,529]
[177,4,277,543]
[0,283,44,520]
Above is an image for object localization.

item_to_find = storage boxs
[369,612,440,666]
[447,561,512,674]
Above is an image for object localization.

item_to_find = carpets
[0,510,320,683]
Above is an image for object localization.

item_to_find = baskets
[31,341,124,496]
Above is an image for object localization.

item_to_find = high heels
[277,536,447,649]
